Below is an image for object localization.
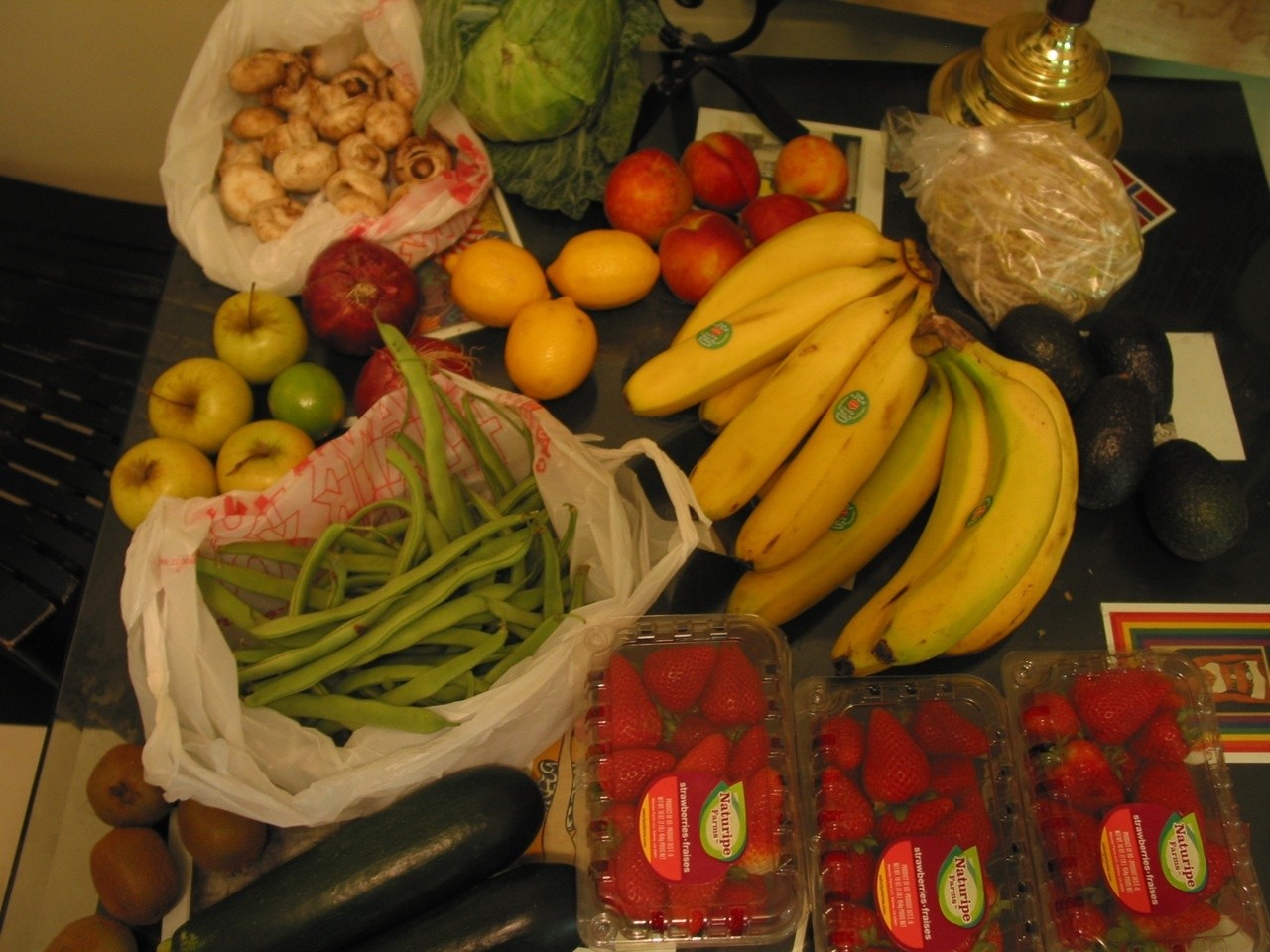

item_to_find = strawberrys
[599,634,799,937]
[1016,665,1235,952]
[808,698,1010,952]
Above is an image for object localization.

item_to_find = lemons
[268,362,346,442]
[442,229,663,400]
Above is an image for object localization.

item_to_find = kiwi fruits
[43,742,271,952]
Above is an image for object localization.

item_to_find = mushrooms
[214,48,450,242]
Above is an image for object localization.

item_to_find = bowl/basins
[566,610,806,944]
[1002,648,1270,952]
[789,671,1041,951]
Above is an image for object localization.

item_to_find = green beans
[192,318,592,740]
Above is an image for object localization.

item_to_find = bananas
[622,212,1078,677]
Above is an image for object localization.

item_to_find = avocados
[998,302,1252,562]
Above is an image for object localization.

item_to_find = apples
[110,281,317,534]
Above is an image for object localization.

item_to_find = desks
[0,0,1269,952]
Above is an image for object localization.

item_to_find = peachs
[603,132,848,304]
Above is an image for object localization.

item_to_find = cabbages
[413,1,668,223]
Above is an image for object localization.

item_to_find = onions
[302,235,476,418]
